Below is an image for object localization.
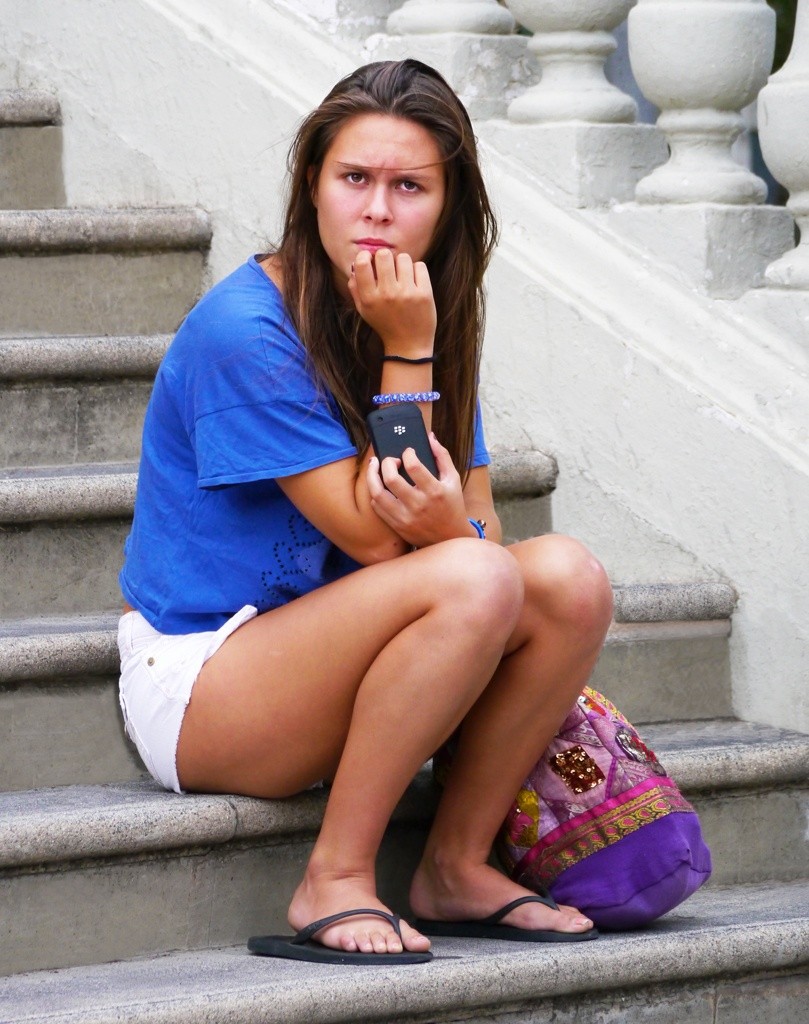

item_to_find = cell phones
[367,404,437,487]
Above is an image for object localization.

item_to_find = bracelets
[470,518,486,540]
[372,392,440,403]
[383,355,433,366]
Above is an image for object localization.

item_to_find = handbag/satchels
[489,686,716,933]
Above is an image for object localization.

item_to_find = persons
[117,60,614,963]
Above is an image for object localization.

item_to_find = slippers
[246,909,432,964]
[408,890,601,944]
